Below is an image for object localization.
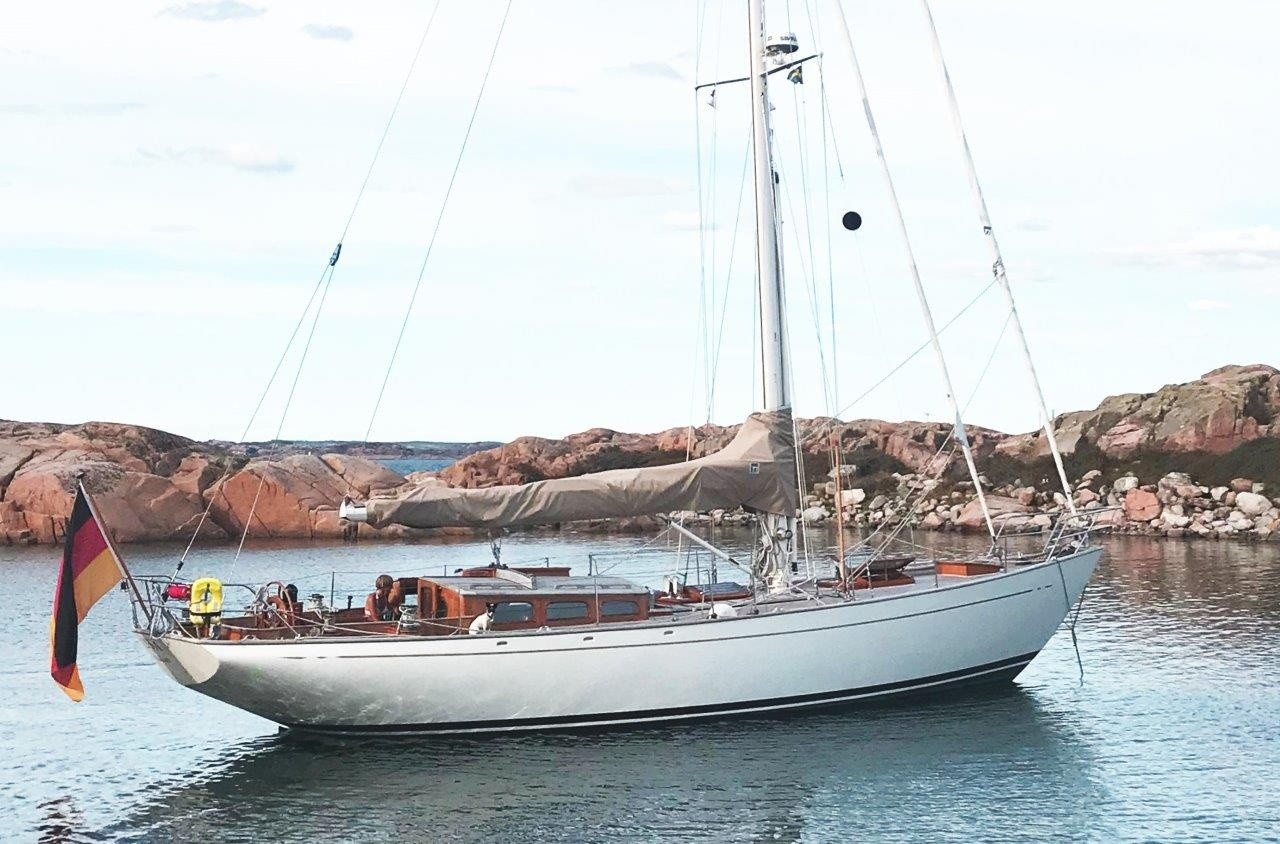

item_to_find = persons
[364,575,394,622]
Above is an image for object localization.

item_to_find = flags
[49,485,124,703]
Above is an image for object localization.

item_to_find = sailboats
[47,0,1113,739]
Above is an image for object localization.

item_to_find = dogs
[469,602,497,634]
